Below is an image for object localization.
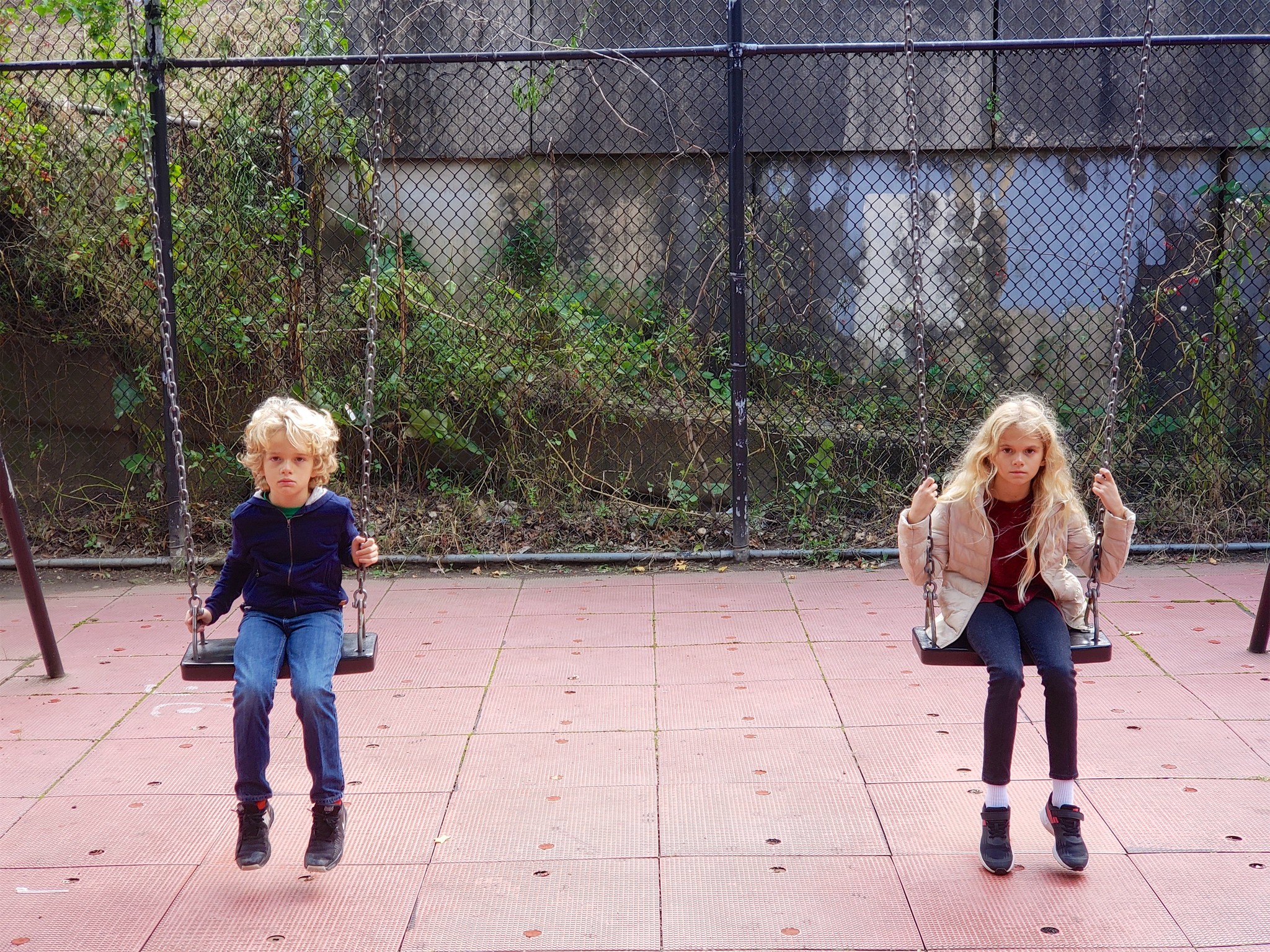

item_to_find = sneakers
[978,802,1015,875]
[1040,791,1089,873]
[235,800,274,870]
[303,800,347,872]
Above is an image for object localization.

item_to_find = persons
[186,396,377,872]
[898,395,1135,876]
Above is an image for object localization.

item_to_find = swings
[123,0,389,679]
[903,1,1155,667]
